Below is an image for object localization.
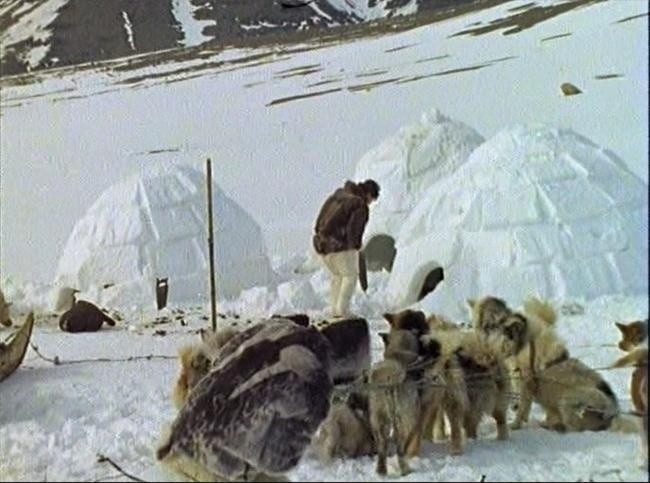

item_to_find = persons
[311,178,381,318]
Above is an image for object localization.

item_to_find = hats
[358,179,380,198]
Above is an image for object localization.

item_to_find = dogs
[0,289,13,326]
[501,297,638,435]
[612,318,649,416]
[310,295,529,476]
